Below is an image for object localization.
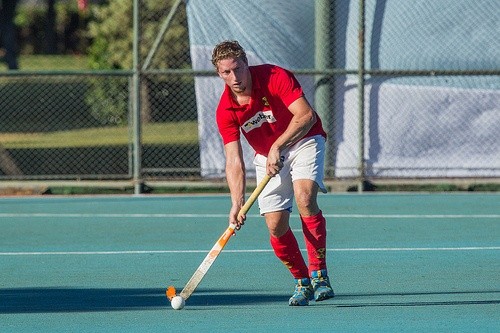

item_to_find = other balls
[171,296,185,310]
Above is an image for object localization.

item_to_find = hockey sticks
[166,155,285,301]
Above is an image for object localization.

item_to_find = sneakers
[310,269,335,302]
[288,278,313,306]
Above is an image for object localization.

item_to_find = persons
[212,40,335,306]
